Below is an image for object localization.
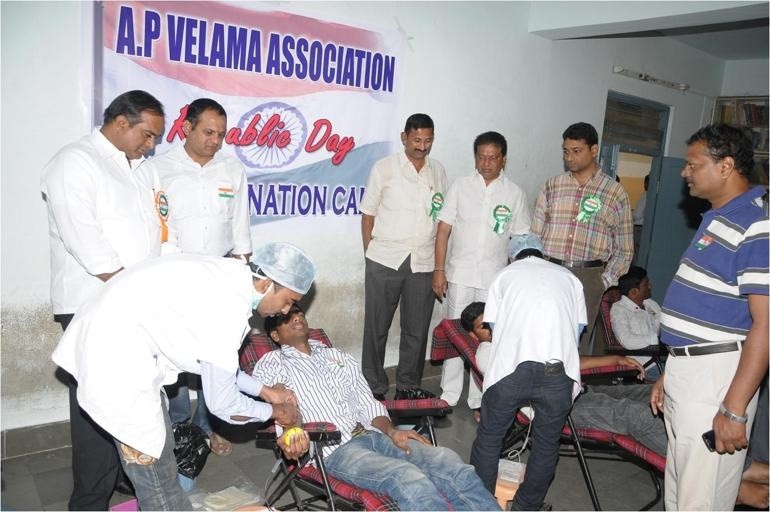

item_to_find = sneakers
[395,389,439,401]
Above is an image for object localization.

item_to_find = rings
[302,449,308,453]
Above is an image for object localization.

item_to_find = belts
[544,257,602,267]
[666,341,746,356]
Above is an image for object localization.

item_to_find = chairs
[237,328,454,511]
[430,317,670,511]
[602,288,669,387]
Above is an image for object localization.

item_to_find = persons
[633,175,650,255]
[40,90,167,511]
[462,301,769,508]
[148,98,252,455]
[532,122,634,357]
[360,113,447,400]
[51,241,316,511]
[253,304,502,511]
[433,131,532,423]
[652,124,769,511]
[610,265,666,380]
[469,233,588,511]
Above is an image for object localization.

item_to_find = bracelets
[434,268,445,271]
[720,403,749,423]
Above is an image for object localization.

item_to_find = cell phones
[702,429,716,452]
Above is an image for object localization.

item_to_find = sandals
[207,430,234,457]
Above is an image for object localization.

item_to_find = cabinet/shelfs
[710,95,769,206]
